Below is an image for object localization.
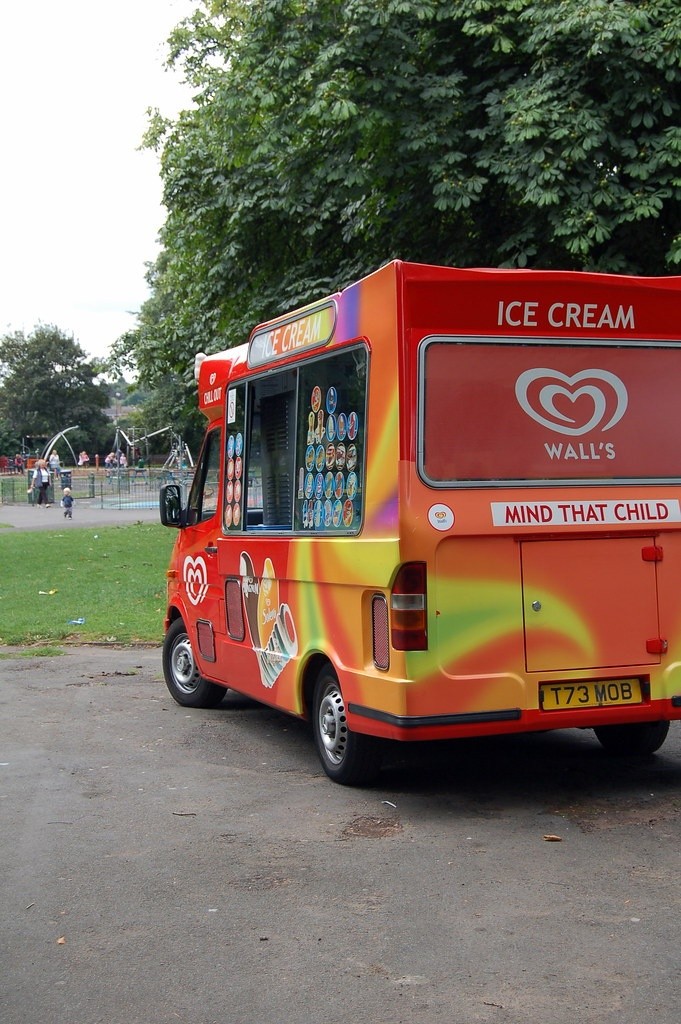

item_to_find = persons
[60,487,74,520]
[132,457,146,481]
[176,459,187,487]
[80,450,89,468]
[106,452,115,477]
[49,449,60,481]
[0,454,8,472]
[31,459,52,508]
[15,454,23,474]
[120,453,127,480]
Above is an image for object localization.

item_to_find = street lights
[115,392,121,423]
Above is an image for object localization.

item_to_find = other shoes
[45,504,51,508]
[37,504,42,509]
[62,515,66,519]
[68,516,72,519]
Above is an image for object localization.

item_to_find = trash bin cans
[60,471,72,490]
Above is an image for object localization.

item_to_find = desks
[109,467,148,484]
[169,469,194,485]
[4,466,15,475]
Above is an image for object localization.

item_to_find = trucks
[159,259,681,785]
